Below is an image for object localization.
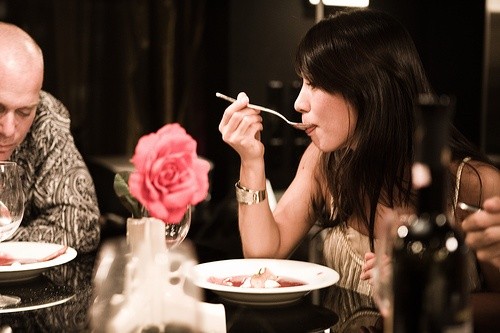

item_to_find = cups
[0,162,24,242]
[141,204,192,252]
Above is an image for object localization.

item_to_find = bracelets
[235,181,267,205]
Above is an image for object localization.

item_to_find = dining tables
[0,249,339,333]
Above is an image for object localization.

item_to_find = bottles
[390,166,474,333]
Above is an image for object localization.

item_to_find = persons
[219,8,500,333]
[462,195,500,270]
[0,20,102,256]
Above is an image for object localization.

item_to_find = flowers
[114,124,214,224]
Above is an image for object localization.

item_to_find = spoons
[216,93,315,131]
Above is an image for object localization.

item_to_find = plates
[0,242,78,283]
[185,259,340,304]
